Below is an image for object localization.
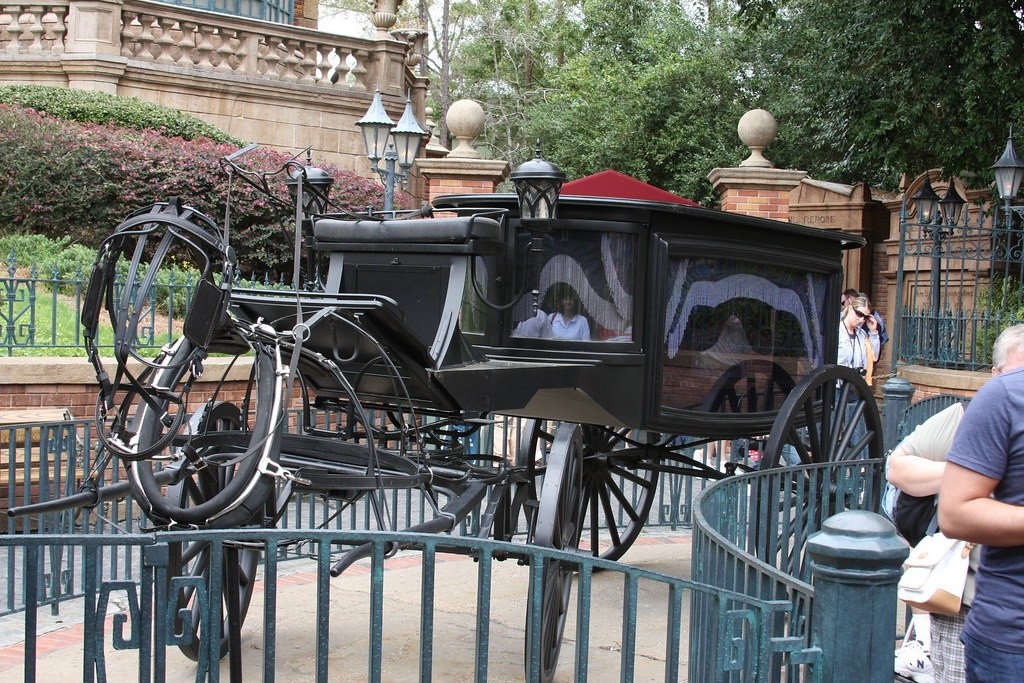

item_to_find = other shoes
[720,461,744,475]
[706,460,716,470]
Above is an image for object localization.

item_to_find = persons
[548,292,591,341]
[692,287,890,474]
[937,367,1024,683]
[886,323,1024,683]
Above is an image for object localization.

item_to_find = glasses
[853,307,871,320]
[841,299,848,306]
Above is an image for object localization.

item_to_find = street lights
[910,171,968,360]
[285,148,337,293]
[356,88,426,220]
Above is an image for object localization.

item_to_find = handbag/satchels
[897,530,973,615]
[879,401,971,547]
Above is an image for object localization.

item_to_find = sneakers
[894,639,935,683]
